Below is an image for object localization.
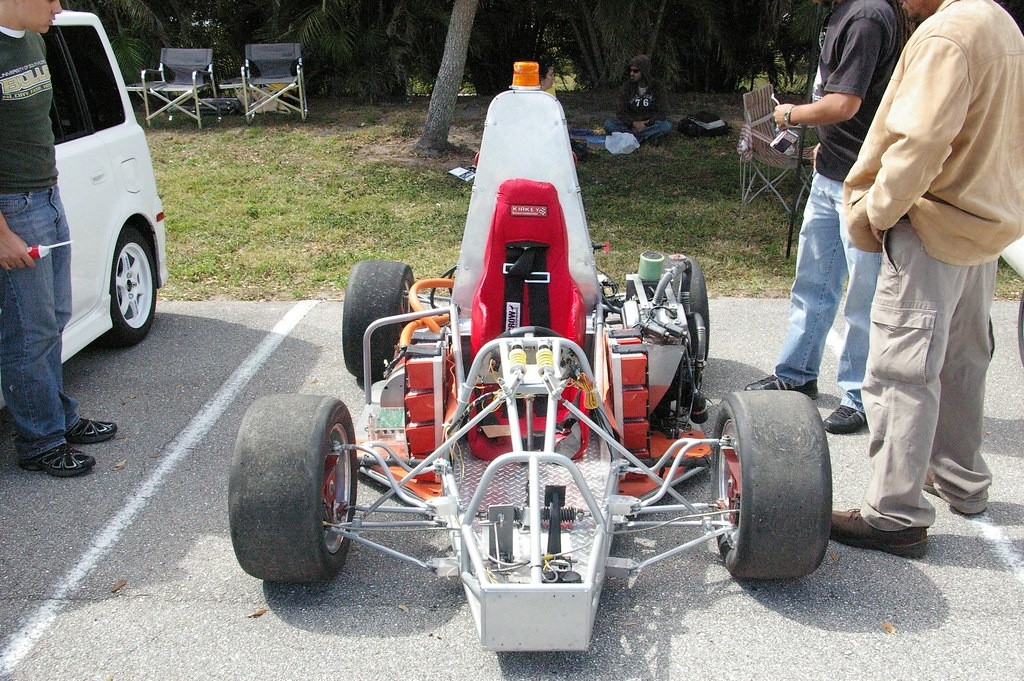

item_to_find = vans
[0,7,169,417]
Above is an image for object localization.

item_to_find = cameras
[770,129,798,154]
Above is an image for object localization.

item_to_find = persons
[537,60,587,160]
[0,0,118,477]
[826,0,1024,556]
[746,0,914,434]
[603,54,673,144]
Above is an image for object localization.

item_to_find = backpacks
[678,111,733,137]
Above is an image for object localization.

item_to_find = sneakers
[64,418,117,443]
[19,443,95,476]
[923,469,989,514]
[745,376,819,400]
[824,405,867,433]
[829,511,927,557]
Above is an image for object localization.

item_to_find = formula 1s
[224,57,837,655]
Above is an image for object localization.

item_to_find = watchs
[782,105,800,128]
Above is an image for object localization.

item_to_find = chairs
[465,179,589,460]
[241,43,308,126]
[736,84,816,215]
[141,49,218,128]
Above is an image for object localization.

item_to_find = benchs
[200,98,238,116]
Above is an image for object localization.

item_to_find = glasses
[627,68,641,73]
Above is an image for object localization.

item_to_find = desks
[126,78,161,94]
[220,76,250,91]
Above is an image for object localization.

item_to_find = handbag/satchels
[605,132,641,154]
